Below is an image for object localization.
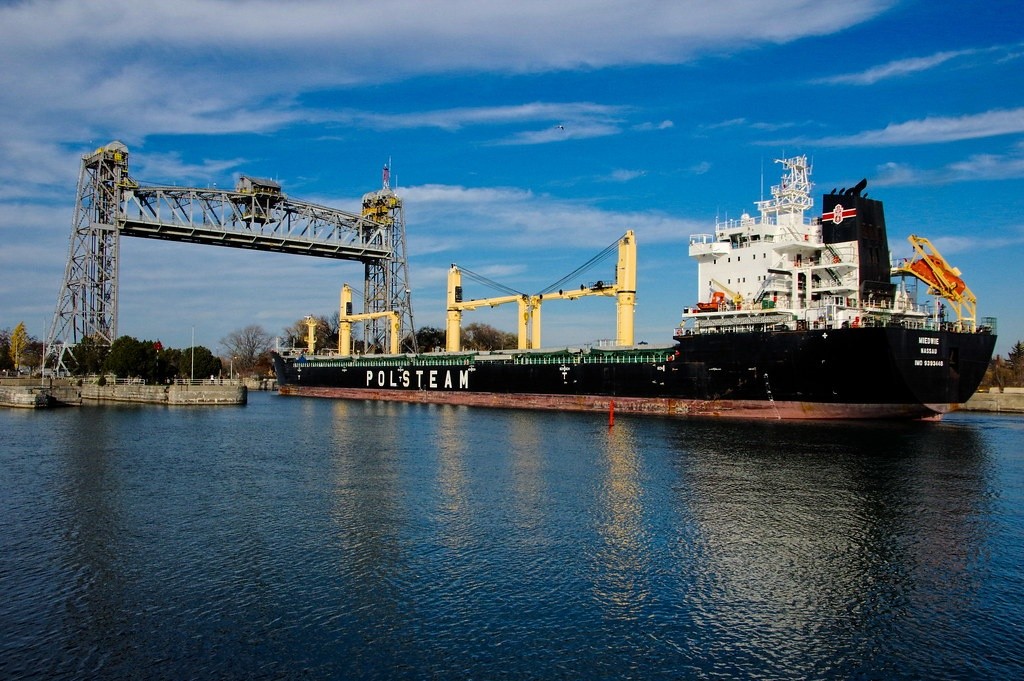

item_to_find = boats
[270,156,998,425]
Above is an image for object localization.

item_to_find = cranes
[709,277,743,305]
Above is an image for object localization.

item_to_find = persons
[210,374,214,385]
[164,386,170,402]
[219,375,224,386]
